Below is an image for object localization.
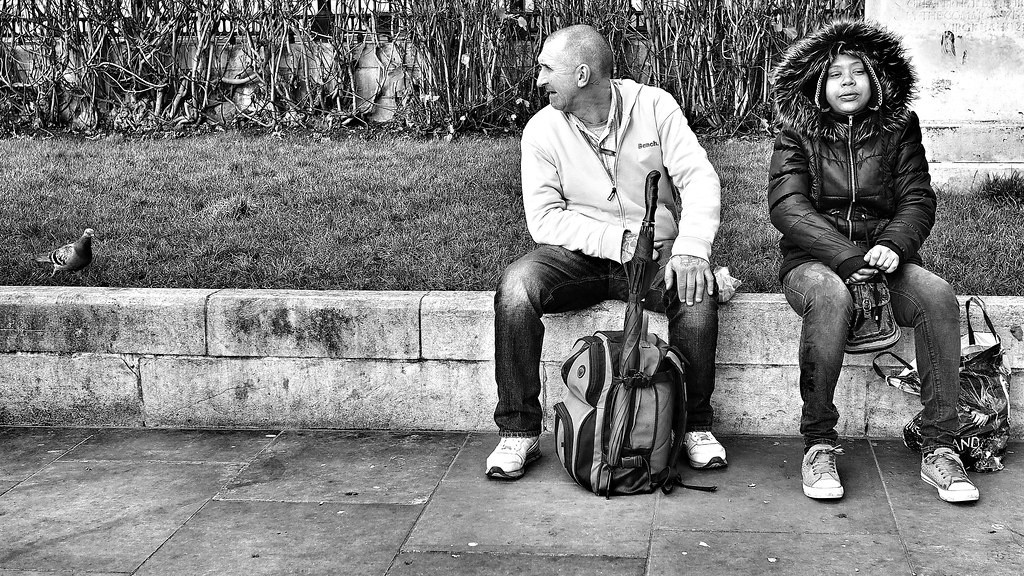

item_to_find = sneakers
[920,448,980,502]
[800,444,845,499]
[683,430,729,471]
[485,435,543,480]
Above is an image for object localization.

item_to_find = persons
[484,24,729,479]
[767,20,979,503]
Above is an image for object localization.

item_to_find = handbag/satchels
[843,273,901,354]
[872,297,1012,472]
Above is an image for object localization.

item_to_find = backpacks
[554,314,717,500]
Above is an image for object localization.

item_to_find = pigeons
[36,228,95,279]
